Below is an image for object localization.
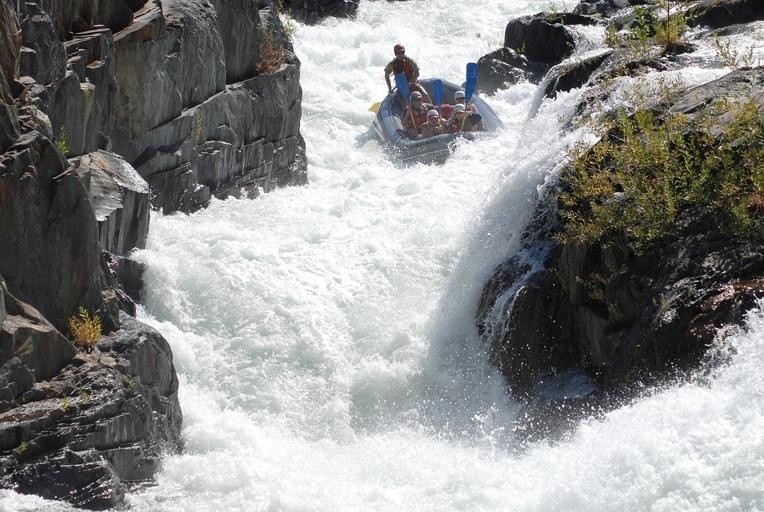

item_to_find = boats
[377,79,504,167]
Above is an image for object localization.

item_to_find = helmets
[455,91,465,101]
[394,44,405,56]
[409,91,423,103]
[426,110,438,121]
[453,104,465,113]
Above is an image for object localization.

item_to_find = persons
[401,90,488,140]
[384,44,432,110]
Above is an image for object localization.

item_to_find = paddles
[432,80,442,118]
[368,87,397,114]
[395,72,418,134]
[462,63,478,130]
